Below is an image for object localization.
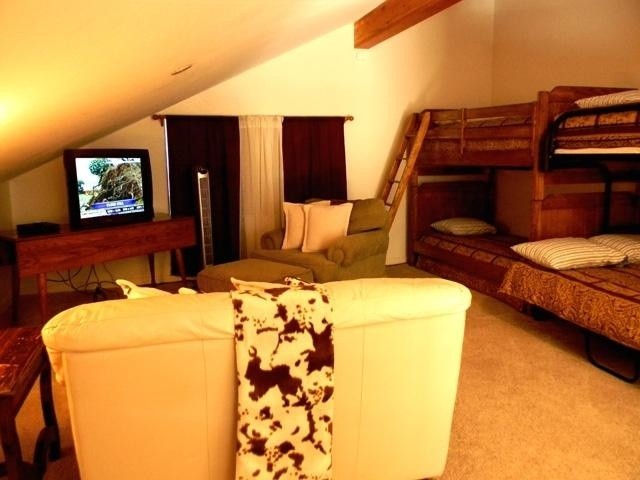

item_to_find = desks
[0,327,62,480]
[0,212,198,323]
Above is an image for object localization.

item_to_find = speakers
[193,165,214,269]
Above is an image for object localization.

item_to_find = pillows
[112,277,264,299]
[280,198,354,255]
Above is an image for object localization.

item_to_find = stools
[195,257,314,295]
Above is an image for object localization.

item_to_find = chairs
[249,196,390,283]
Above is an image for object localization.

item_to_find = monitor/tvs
[63,149,154,232]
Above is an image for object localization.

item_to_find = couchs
[40,276,474,480]
